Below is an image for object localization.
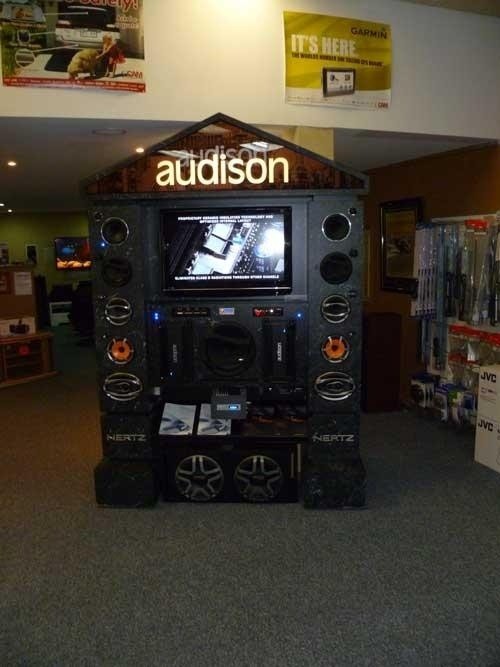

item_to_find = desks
[49,301,74,326]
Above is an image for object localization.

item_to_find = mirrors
[380,196,424,295]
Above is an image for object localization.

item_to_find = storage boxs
[471,416,500,473]
[474,363,500,425]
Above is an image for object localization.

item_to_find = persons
[96,33,125,75]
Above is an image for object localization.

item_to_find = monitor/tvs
[54,237,92,271]
[143,196,313,303]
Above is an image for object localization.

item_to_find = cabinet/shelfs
[0,330,61,388]
